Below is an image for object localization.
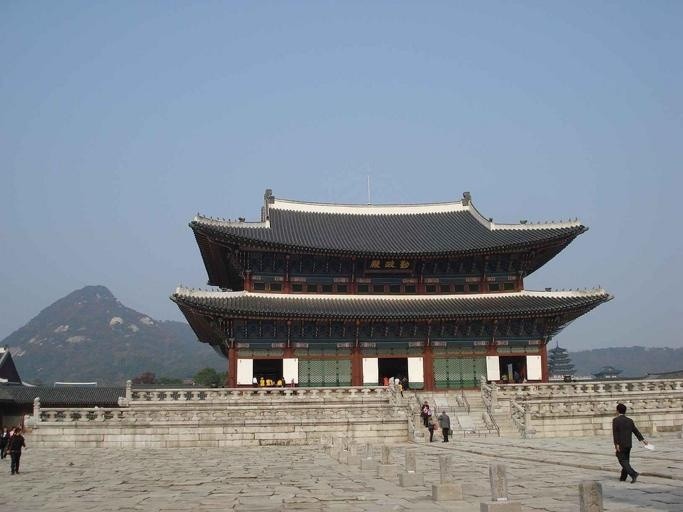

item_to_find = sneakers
[631,473,639,483]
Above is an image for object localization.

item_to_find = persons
[513,370,519,383]
[426,409,432,427]
[251,374,286,395]
[438,409,450,443]
[611,403,648,484]
[0,422,27,476]
[502,373,507,384]
[381,374,408,396]
[428,417,434,442]
[420,401,430,426]
